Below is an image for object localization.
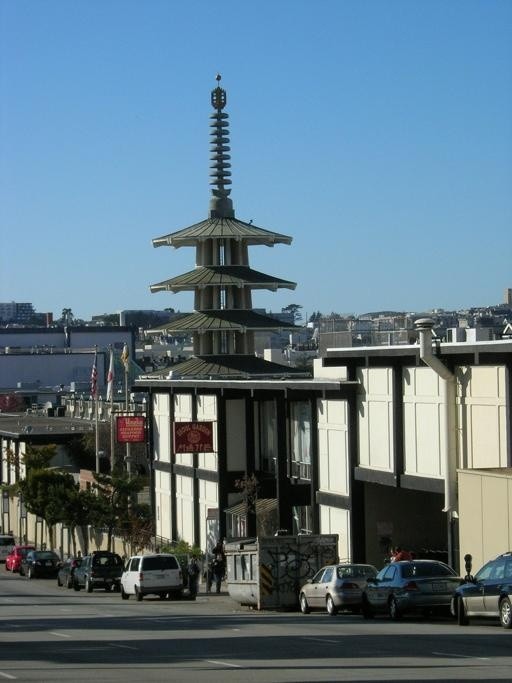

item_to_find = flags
[91,354,97,400]
[121,345,129,372]
[106,351,114,401]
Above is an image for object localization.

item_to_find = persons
[395,546,408,560]
[189,552,225,593]
[390,547,397,562]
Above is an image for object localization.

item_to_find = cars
[0,535,184,602]
[298,551,512,628]
